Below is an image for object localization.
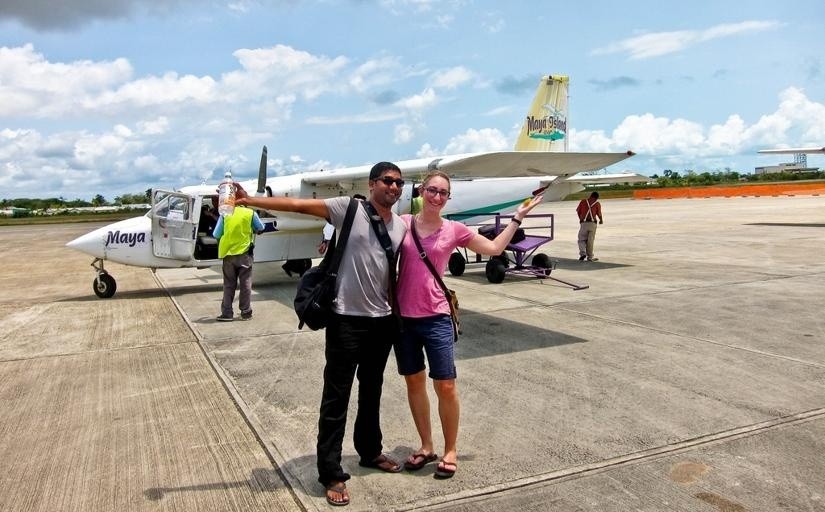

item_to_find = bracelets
[210,213,213,217]
[512,215,522,226]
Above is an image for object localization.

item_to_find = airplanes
[63,72,665,301]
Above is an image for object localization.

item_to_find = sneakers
[579,255,598,262]
[216,314,252,321]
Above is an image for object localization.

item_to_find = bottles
[217,171,236,218]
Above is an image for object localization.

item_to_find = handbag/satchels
[296,263,337,330]
[445,289,462,342]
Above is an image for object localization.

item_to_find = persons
[412,183,425,216]
[214,162,409,505]
[211,202,266,322]
[199,196,220,235]
[575,192,604,262]
[394,170,543,478]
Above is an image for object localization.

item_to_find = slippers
[358,454,403,472]
[434,455,458,479]
[404,452,438,471]
[325,479,350,506]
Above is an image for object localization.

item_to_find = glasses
[423,187,451,200]
[374,176,404,188]
